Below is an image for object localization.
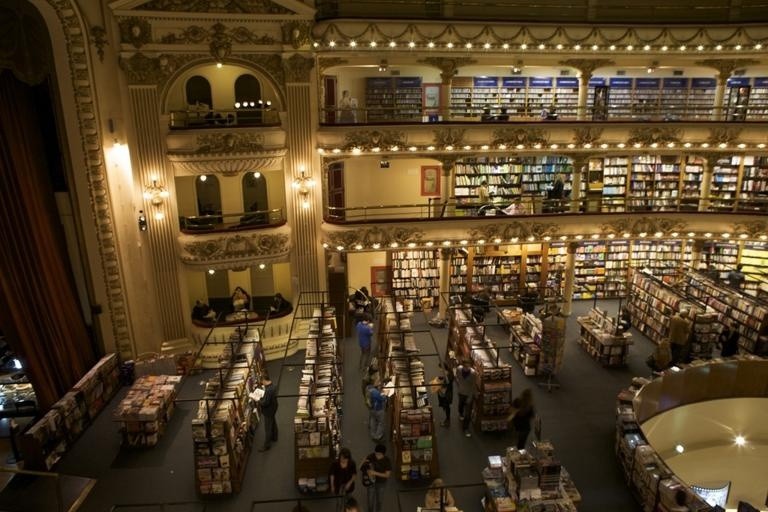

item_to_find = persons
[367,374,390,441]
[356,314,375,372]
[345,497,361,512]
[361,444,392,512]
[361,359,384,392]
[257,374,278,451]
[194,300,215,321]
[340,90,355,123]
[274,294,286,313]
[331,448,357,511]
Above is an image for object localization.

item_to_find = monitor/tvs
[209,296,234,315]
[252,294,274,312]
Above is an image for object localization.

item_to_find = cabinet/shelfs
[392,241,767,309]
[572,308,634,369]
[189,324,270,498]
[497,306,567,390]
[636,308,767,358]
[614,357,766,512]
[377,293,441,490]
[478,438,583,510]
[21,351,125,473]
[452,155,768,216]
[292,306,346,497]
[363,84,767,124]
[441,306,514,436]
[0,370,39,418]
[111,373,183,446]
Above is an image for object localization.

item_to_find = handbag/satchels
[362,463,376,486]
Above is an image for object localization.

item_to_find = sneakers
[258,446,268,453]
[439,416,472,438]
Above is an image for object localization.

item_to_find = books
[380,373,581,511]
[190,326,265,494]
[450,86,768,121]
[616,355,767,512]
[453,155,768,215]
[28,355,181,450]
[364,86,424,121]
[379,238,580,376]
[204,311,218,320]
[578,236,767,367]
[294,305,344,494]
